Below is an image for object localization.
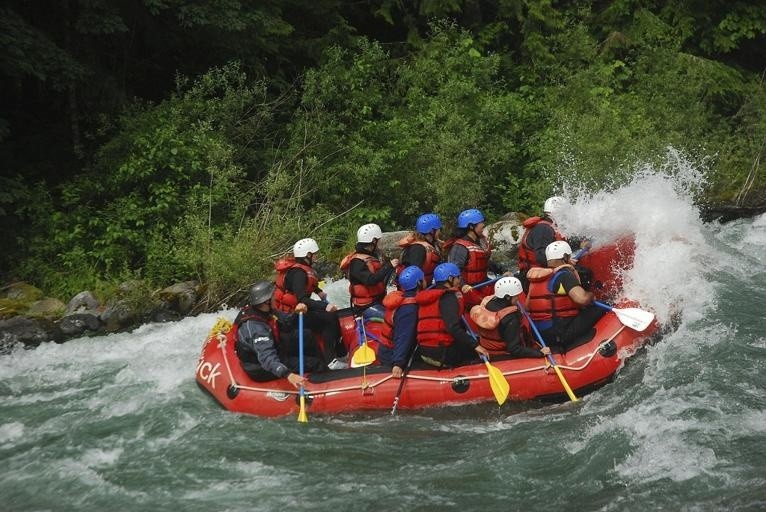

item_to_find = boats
[189,229,660,412]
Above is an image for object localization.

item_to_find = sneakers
[326,353,351,369]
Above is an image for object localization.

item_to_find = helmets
[398,265,424,291]
[544,197,570,215]
[432,263,462,282]
[416,214,442,235]
[494,276,524,299]
[292,238,319,259]
[248,279,278,307]
[356,224,382,243]
[545,240,573,261]
[456,209,484,228]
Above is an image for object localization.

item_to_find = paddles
[594,301,655,332]
[461,315,510,406]
[297,310,309,423]
[349,315,376,368]
[515,298,579,403]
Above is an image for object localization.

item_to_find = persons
[272,238,350,370]
[525,240,607,345]
[236,282,327,388]
[339,223,397,322]
[517,197,593,294]
[418,263,489,366]
[378,265,426,378]
[445,209,511,306]
[399,212,443,283]
[469,276,552,359]
